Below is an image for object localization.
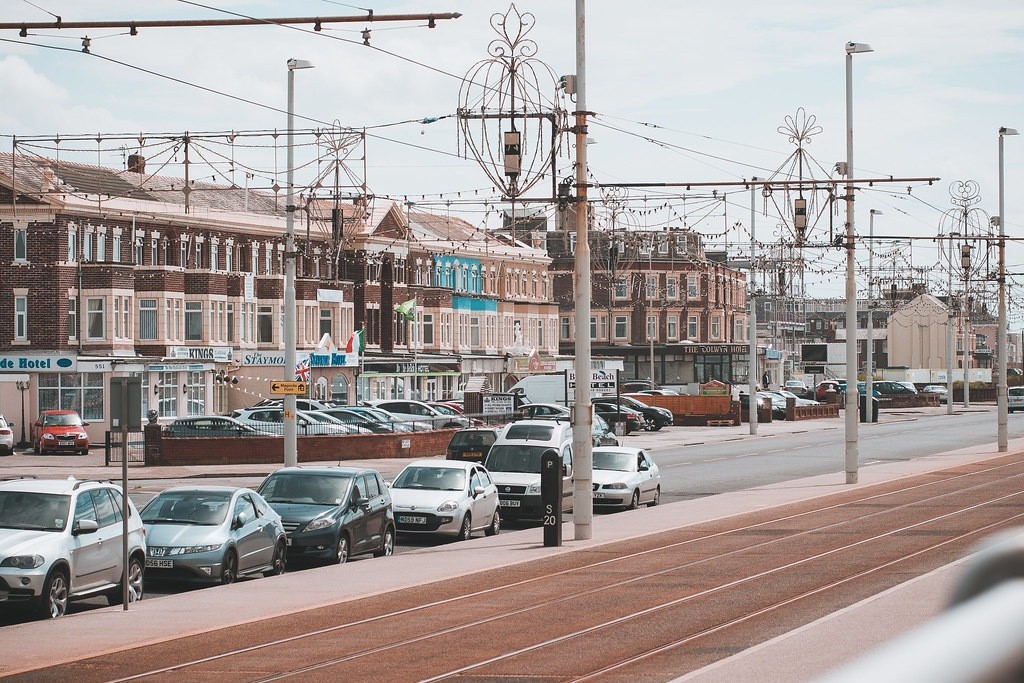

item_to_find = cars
[591,446,661,510]
[738,389,821,421]
[623,380,691,396]
[254,465,397,565]
[0,415,15,455]
[924,385,948,404]
[782,380,809,399]
[446,428,502,465]
[591,395,674,446]
[1007,386,1024,413]
[160,398,488,438]
[32,410,91,456]
[815,378,918,402]
[137,485,288,586]
[386,458,501,541]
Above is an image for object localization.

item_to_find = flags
[295,359,310,382]
[347,330,364,353]
[395,300,415,321]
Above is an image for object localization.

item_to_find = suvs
[0,472,148,620]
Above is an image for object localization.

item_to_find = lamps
[154,384,158,395]
[110,360,117,370]
[183,384,187,394]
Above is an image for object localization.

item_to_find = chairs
[169,500,196,521]
[414,470,463,490]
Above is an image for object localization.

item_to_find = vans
[483,414,576,520]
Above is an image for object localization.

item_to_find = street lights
[996,126,1021,451]
[749,176,767,435]
[865,209,884,422]
[282,57,315,467]
[844,41,876,485]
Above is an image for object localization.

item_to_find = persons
[763,371,769,388]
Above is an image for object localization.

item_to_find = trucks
[506,375,566,409]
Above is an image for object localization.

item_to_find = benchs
[196,504,229,523]
[706,420,734,427]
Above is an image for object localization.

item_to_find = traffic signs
[269,381,308,394]
